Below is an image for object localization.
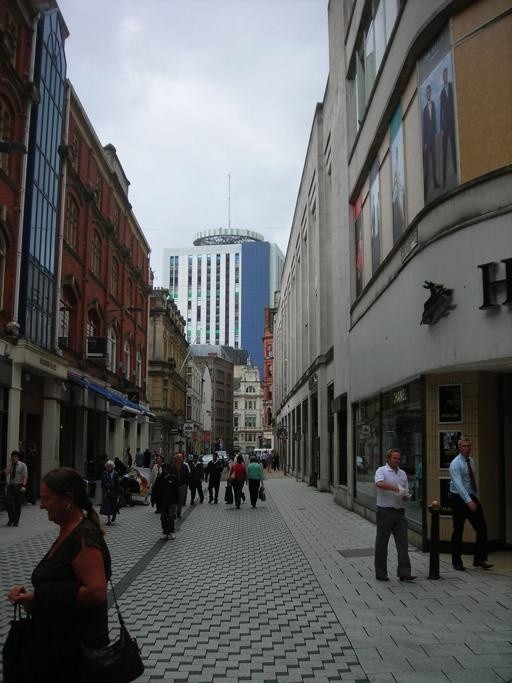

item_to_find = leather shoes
[476,562,494,568]
[380,577,389,581]
[7,520,19,527]
[400,575,417,581]
[454,565,466,571]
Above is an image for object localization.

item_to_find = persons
[101,446,281,540]
[375,447,417,581]
[10,468,110,683]
[1,450,27,525]
[449,436,495,571]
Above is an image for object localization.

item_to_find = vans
[254,448,273,456]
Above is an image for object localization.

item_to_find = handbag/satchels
[3,620,85,683]
[225,487,233,504]
[81,624,144,683]
[228,471,235,480]
[259,487,265,501]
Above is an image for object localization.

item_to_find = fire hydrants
[428,501,442,580]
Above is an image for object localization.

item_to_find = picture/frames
[437,477,452,514]
[438,430,464,471]
[437,382,464,425]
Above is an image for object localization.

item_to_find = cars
[202,451,229,469]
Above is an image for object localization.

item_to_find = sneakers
[191,499,217,505]
[106,516,115,525]
[160,532,176,540]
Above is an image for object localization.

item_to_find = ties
[11,463,17,479]
[465,458,476,493]
